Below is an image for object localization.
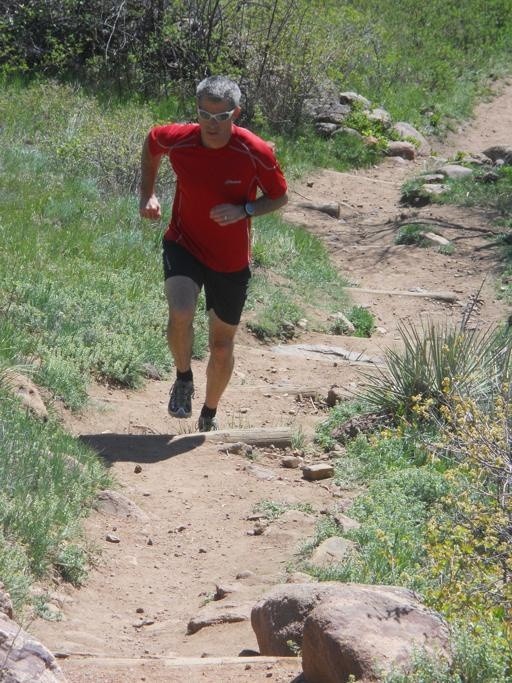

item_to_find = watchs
[244,201,256,217]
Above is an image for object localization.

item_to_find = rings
[225,215,227,221]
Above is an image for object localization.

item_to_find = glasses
[195,98,237,123]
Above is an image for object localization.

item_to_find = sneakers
[167,377,195,417]
[198,413,214,432]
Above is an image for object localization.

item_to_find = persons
[137,73,288,433]
